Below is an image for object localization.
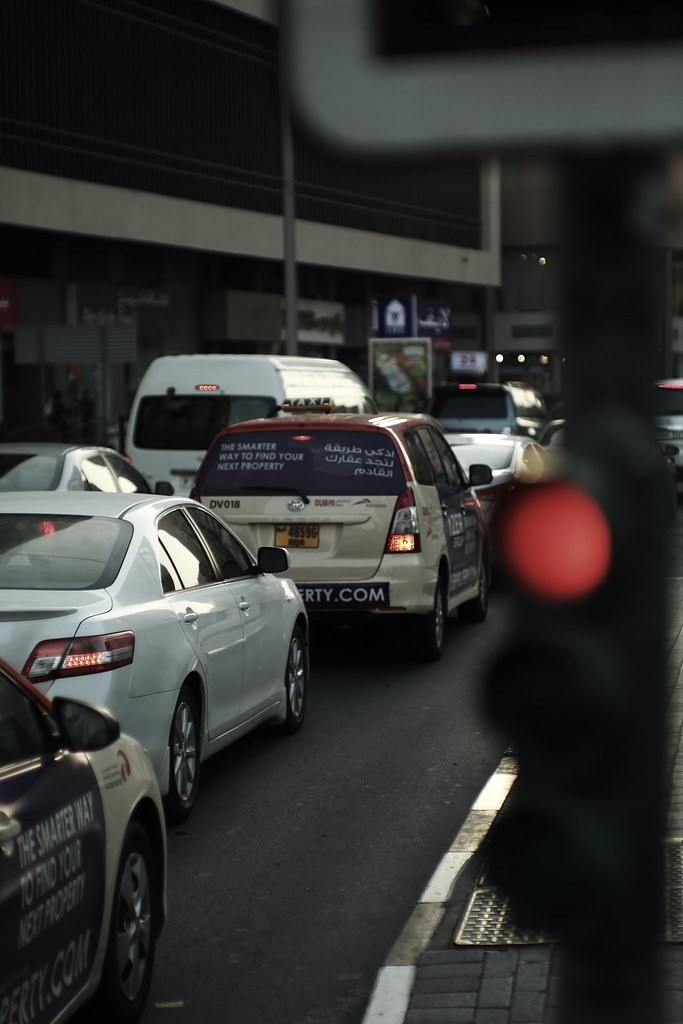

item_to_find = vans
[426,381,550,438]
[657,378,683,468]
[124,353,379,504]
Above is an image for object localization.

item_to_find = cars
[0,491,313,821]
[448,420,566,479]
[0,659,169,1024]
[0,443,173,551]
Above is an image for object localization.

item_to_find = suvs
[188,414,494,660]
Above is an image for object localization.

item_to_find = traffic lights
[468,463,644,951]
